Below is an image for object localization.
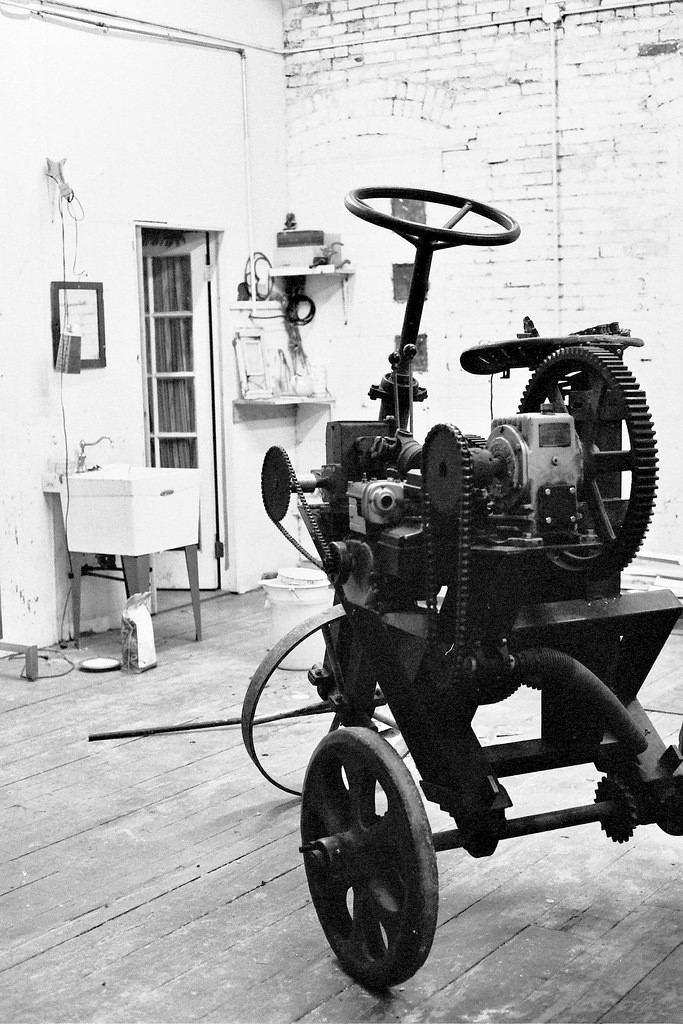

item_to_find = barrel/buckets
[259,568,336,670]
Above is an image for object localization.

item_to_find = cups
[293,377,311,397]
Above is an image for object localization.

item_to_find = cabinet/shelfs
[234,228,355,407]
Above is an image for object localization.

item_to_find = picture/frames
[52,281,106,369]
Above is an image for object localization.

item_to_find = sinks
[60,463,200,557]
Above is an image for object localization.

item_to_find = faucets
[76,436,114,474]
